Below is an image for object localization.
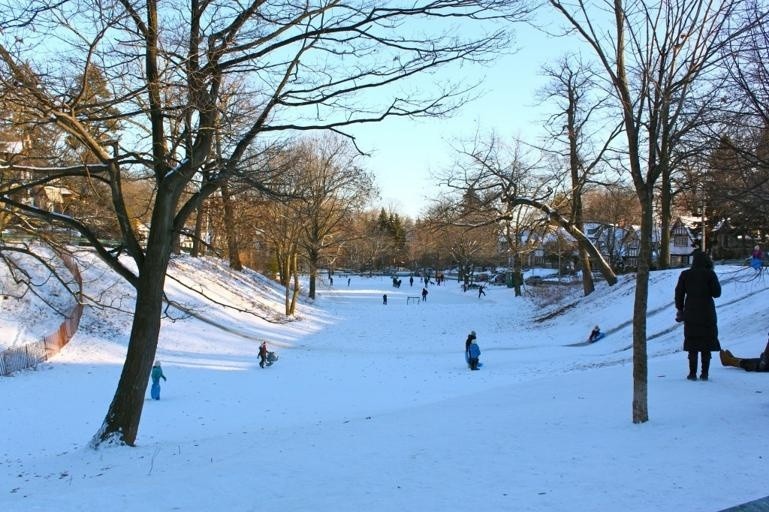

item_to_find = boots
[720,348,743,368]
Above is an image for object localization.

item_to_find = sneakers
[259,362,264,368]
[700,374,708,381]
[687,373,697,381]
[471,367,480,370]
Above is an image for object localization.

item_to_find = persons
[465,331,483,367]
[468,336,480,370]
[589,324,604,343]
[720,330,768,372]
[478,285,486,298]
[257,339,269,368]
[410,276,413,286]
[674,250,722,380]
[421,288,428,301]
[151,361,166,399]
